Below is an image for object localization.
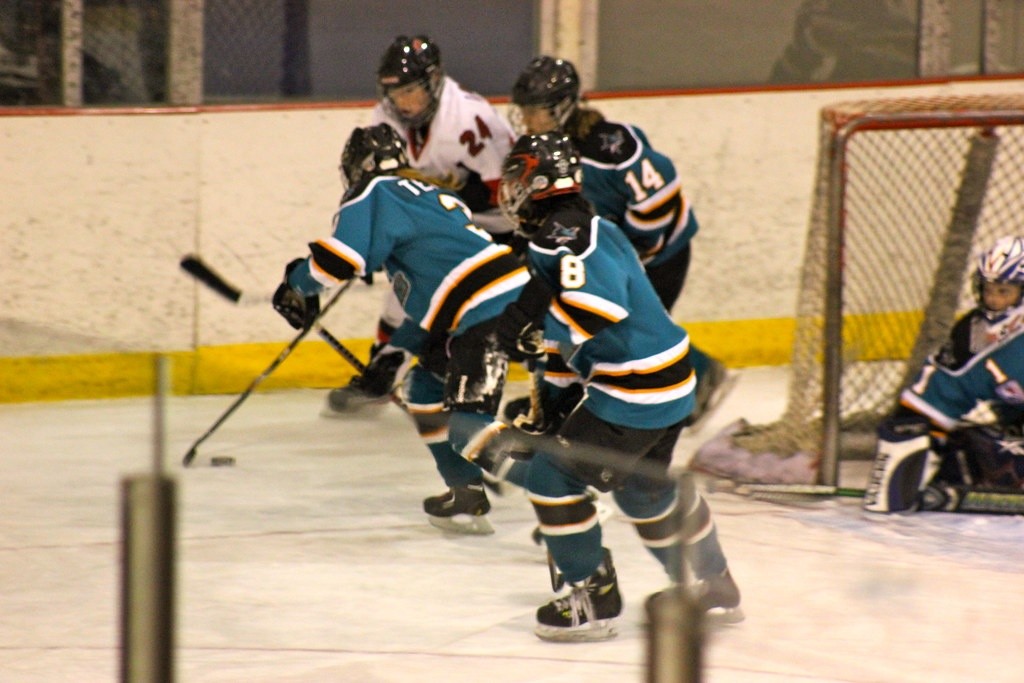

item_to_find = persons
[497,54,742,642]
[272,34,539,535]
[863,236,1024,513]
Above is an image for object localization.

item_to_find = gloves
[272,284,321,330]
[496,300,547,361]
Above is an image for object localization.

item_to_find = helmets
[378,35,444,127]
[497,131,583,238]
[513,56,579,136]
[341,122,406,190]
[971,236,1024,342]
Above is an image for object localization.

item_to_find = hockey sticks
[178,252,417,419]
[702,479,867,500]
[515,333,614,546]
[178,274,358,472]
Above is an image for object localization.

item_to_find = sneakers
[534,547,623,642]
[647,552,746,624]
[422,483,494,536]
[320,372,392,419]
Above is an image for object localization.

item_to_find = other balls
[207,454,236,468]
